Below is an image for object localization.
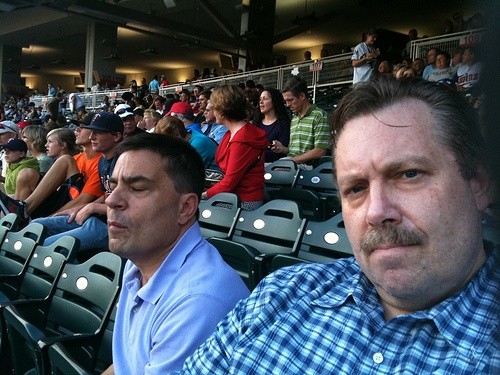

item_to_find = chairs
[0,212,120,375]
[198,159,355,293]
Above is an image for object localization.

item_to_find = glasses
[115,107,133,115]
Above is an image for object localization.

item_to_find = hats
[114,104,134,119]
[69,111,95,126]
[0,120,19,134]
[0,138,27,152]
[165,101,192,118]
[80,111,124,134]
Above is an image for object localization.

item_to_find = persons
[99,132,251,375]
[0,0,500,302]
[164,74,500,375]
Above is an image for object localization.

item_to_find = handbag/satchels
[203,164,225,189]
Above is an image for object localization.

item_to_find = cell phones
[269,144,275,147]
